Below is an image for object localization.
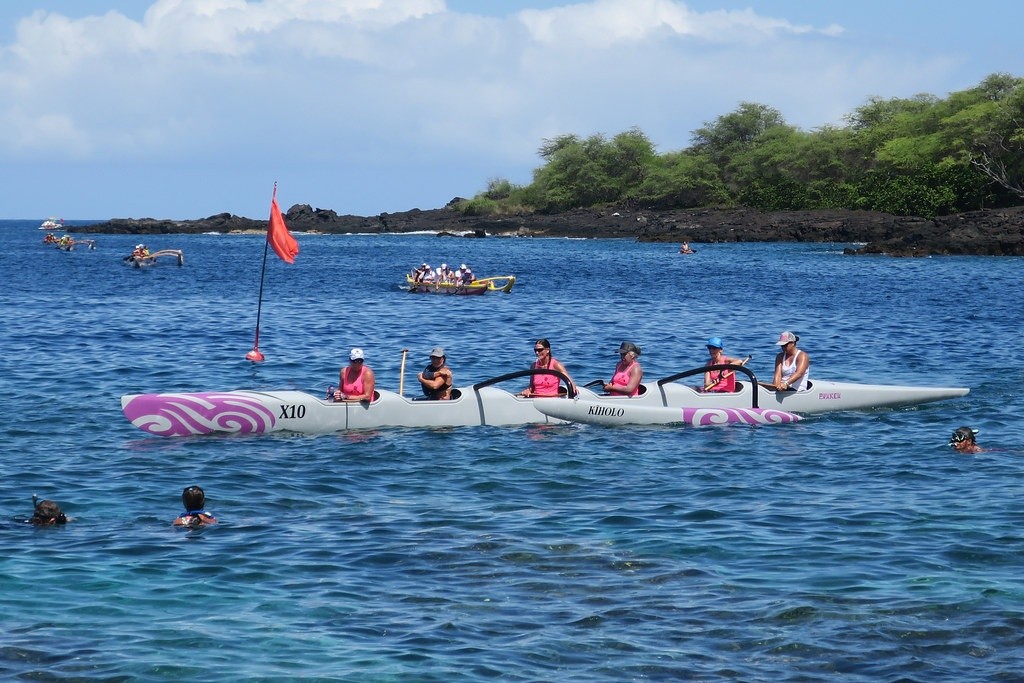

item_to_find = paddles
[758,381,778,388]
[400,348,409,396]
[514,392,567,397]
[704,354,752,390]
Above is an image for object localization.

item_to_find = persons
[170,486,219,527]
[681,240,693,253]
[44,232,73,247]
[417,347,453,400]
[29,494,77,526]
[774,329,812,393]
[333,348,375,403]
[704,337,743,393]
[521,338,580,397]
[948,427,983,450]
[133,243,151,259]
[604,342,643,396]
[414,262,477,290]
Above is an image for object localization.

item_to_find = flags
[266,186,299,264]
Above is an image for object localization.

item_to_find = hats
[441,263,447,270]
[773,331,797,346]
[421,263,427,266]
[465,268,471,273]
[348,348,364,360]
[614,342,641,355]
[429,347,445,357]
[460,263,467,269]
[705,337,723,350]
[424,265,431,269]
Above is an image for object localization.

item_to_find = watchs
[784,381,789,385]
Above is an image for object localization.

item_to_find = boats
[473,274,516,292]
[534,363,971,428]
[406,273,489,295]
[681,249,698,254]
[120,374,601,432]
[123,249,184,267]
[38,217,64,231]
[43,238,101,252]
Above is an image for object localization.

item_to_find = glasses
[534,348,544,352]
[31,517,51,526]
[352,359,364,364]
[951,431,976,443]
